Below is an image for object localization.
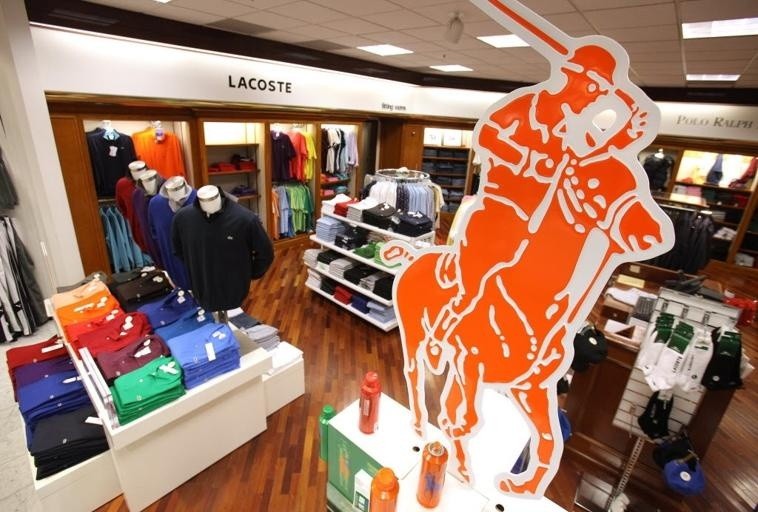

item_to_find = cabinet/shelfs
[18,268,308,512]
[400,115,758,268]
[46,92,382,250]
[304,168,445,335]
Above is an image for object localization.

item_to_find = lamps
[444,11,464,44]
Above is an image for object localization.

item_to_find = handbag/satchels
[664,453,704,496]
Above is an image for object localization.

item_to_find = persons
[115,161,147,254]
[131,169,167,264]
[146,176,200,292]
[169,184,274,313]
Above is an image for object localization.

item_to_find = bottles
[369,468,399,512]
[357,372,382,433]
[416,440,448,509]
[318,405,337,460]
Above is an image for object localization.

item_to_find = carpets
[0,319,53,512]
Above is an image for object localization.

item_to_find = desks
[558,259,742,503]
